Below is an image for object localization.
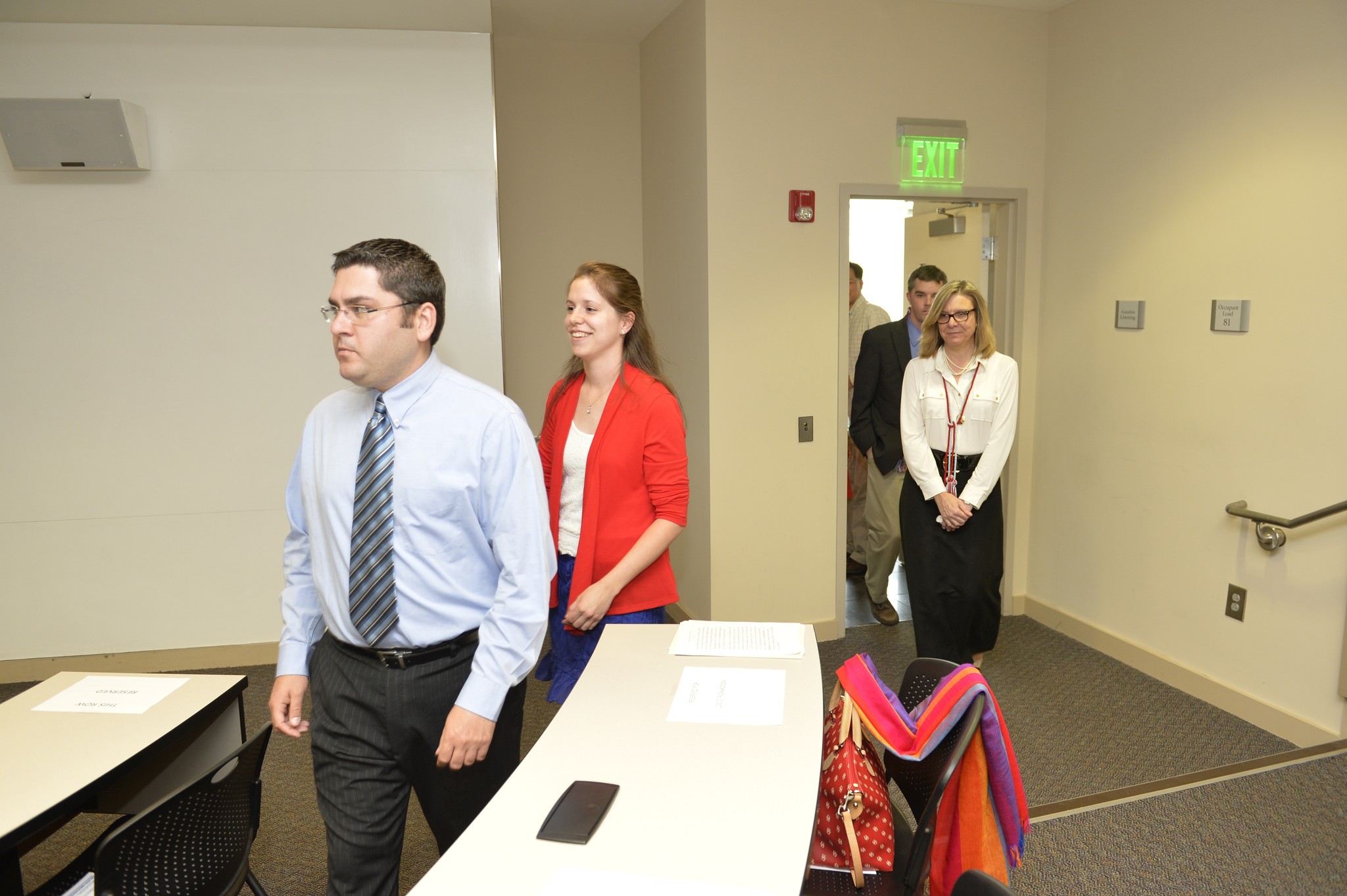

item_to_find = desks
[1,669,258,896]
[406,621,827,896]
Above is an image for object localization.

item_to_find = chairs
[809,656,984,896]
[18,721,275,896]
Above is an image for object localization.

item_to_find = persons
[897,277,1022,680]
[846,265,947,625]
[847,260,892,577]
[269,238,555,895]
[533,262,690,707]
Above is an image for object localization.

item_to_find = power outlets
[1225,583,1249,623]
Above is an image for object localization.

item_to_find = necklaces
[950,390,969,424]
[941,344,979,376]
[585,374,614,415]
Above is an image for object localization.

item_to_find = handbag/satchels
[809,679,894,888]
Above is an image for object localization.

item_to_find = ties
[347,395,400,649]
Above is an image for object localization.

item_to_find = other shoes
[865,585,899,625]
[846,556,868,575]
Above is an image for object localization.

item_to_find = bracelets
[963,500,974,508]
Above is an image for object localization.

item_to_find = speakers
[1,97,151,172]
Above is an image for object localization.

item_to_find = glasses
[320,300,414,324]
[937,308,977,324]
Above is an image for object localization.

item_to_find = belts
[326,630,487,669]
